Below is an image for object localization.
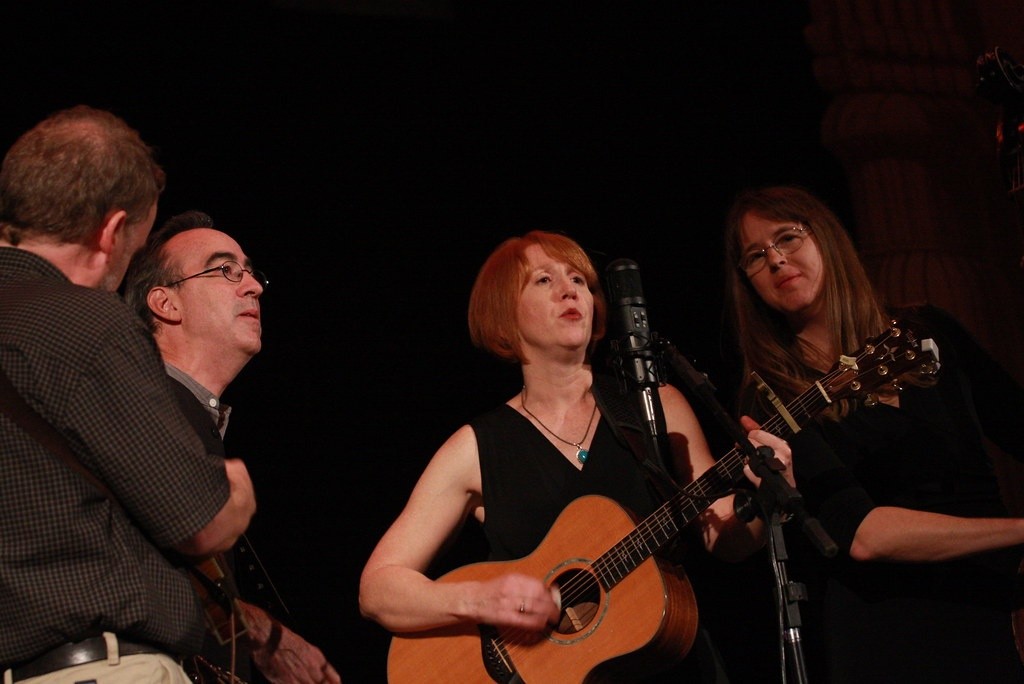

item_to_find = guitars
[193,555,248,649]
[386,317,937,684]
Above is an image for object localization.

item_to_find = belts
[0,632,161,684]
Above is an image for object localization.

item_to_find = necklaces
[520,388,597,463]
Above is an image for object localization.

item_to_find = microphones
[606,253,658,438]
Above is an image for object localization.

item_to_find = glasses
[738,226,809,272]
[164,260,269,290]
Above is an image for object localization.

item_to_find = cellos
[969,44,1024,664]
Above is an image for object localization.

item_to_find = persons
[724,187,1024,684]
[0,106,343,684]
[354,231,795,684]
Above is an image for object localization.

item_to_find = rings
[519,597,527,613]
